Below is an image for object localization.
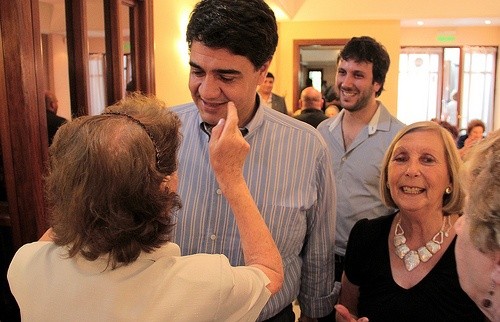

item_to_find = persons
[7,93,285,322]
[256,72,343,129]
[44,92,68,147]
[431,89,487,150]
[165,0,341,322]
[454,125,500,322]
[334,120,491,322]
[316,35,409,322]
[126,81,137,96]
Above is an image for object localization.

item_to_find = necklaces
[393,216,452,272]
